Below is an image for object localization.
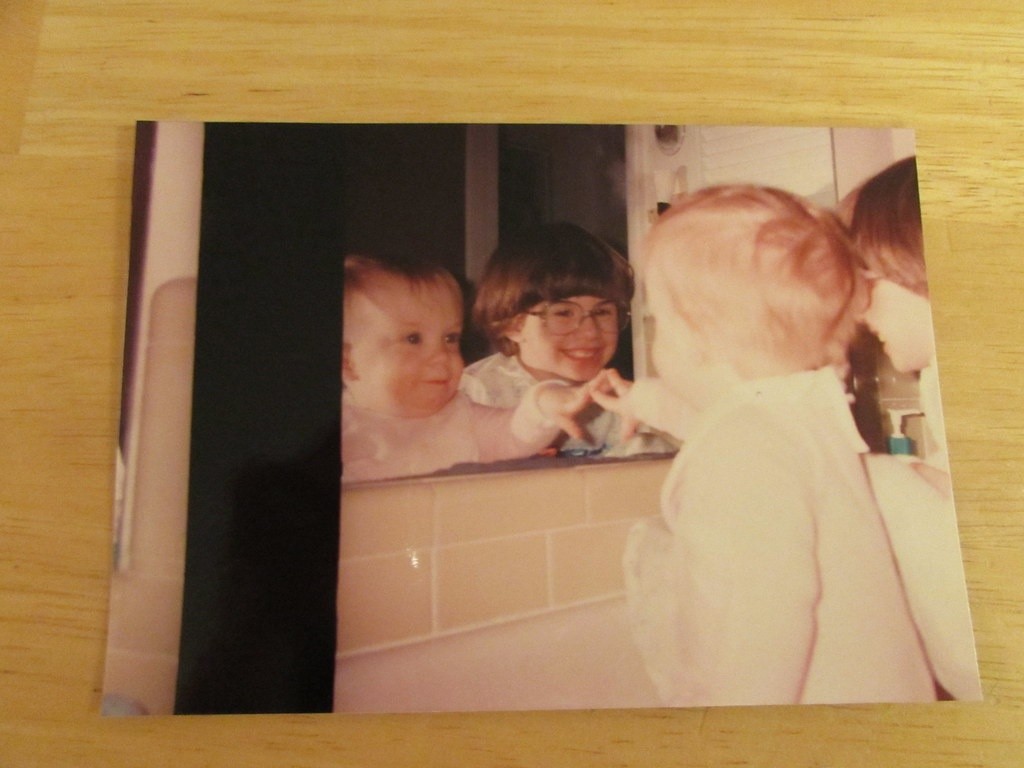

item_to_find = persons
[338,156,982,703]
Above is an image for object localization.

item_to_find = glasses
[521,298,631,336]
